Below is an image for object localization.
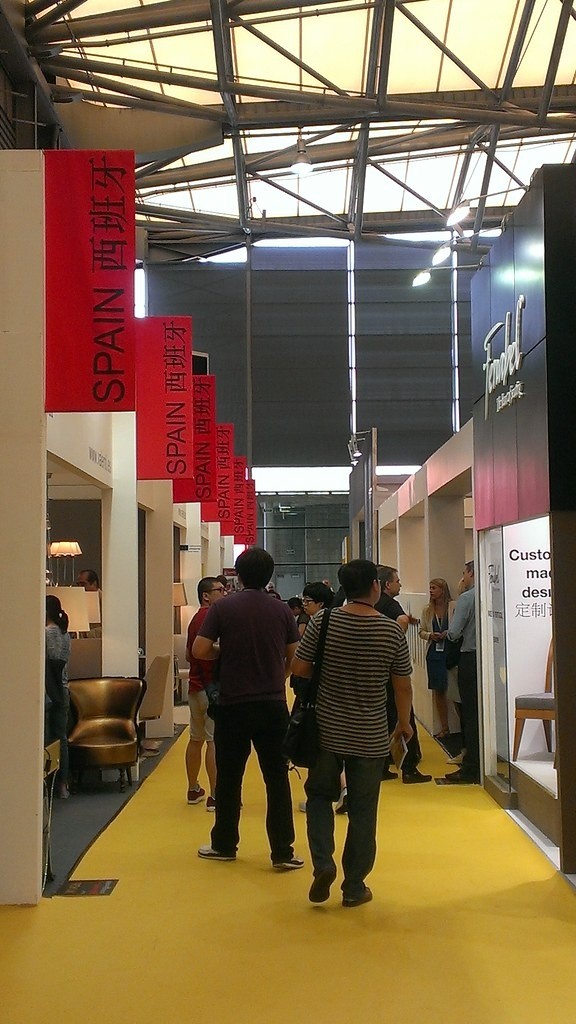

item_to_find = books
[389,728,408,770]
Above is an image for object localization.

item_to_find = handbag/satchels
[287,707,321,767]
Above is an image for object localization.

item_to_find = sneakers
[185,786,244,813]
[273,858,304,870]
[299,787,349,816]
[197,847,238,862]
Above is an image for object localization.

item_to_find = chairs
[140,654,170,756]
[512,634,555,761]
[64,676,148,792]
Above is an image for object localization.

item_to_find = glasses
[201,586,230,595]
[77,581,90,587]
[302,598,314,607]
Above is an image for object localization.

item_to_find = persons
[289,559,413,907]
[76,570,102,605]
[191,547,305,869]
[184,560,477,814]
[46,595,72,799]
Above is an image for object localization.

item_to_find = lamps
[411,183,531,287]
[47,539,100,639]
[291,137,313,175]
[172,582,188,634]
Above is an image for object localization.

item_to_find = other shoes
[59,789,70,799]
[446,751,463,765]
[434,729,451,739]
[445,769,478,782]
[309,866,372,909]
[382,765,431,783]
